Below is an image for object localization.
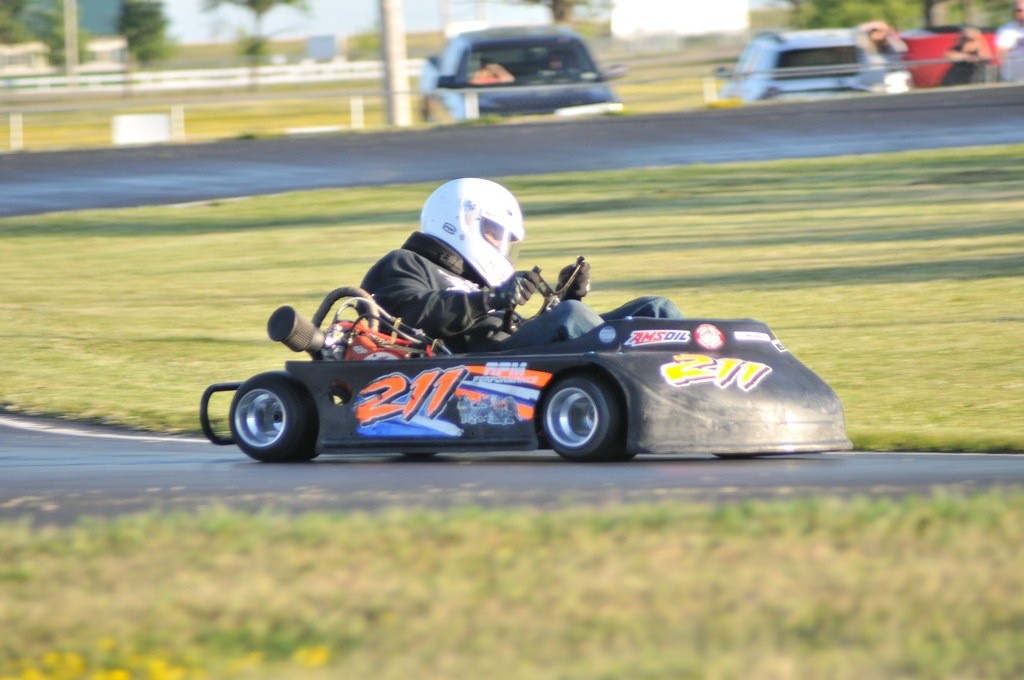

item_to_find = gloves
[496,271,539,306]
[558,262,591,297]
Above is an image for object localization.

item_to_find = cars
[892,24,999,88]
[418,27,627,127]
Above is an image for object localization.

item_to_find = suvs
[710,27,914,102]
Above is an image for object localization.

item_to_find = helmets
[421,177,525,288]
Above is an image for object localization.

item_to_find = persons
[547,52,564,70]
[469,54,516,85]
[356,178,685,352]
[994,1,1024,82]
[854,19,908,95]
[938,24,990,88]
